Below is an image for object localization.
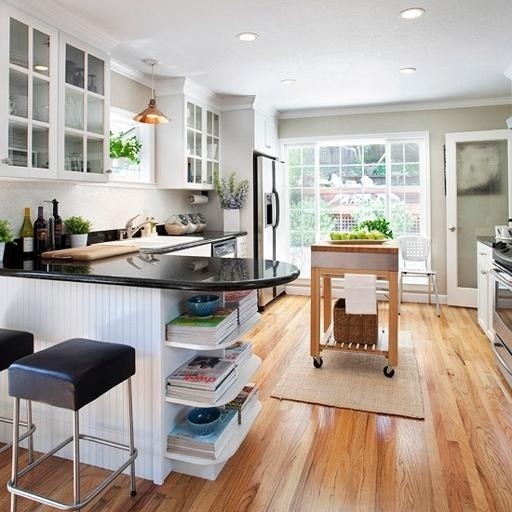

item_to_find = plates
[325,238,389,245]
[8,49,28,69]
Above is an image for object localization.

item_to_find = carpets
[268,327,425,420]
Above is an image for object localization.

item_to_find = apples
[330,230,388,241]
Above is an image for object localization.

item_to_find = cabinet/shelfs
[1,2,111,183]
[156,95,221,190]
[477,236,493,342]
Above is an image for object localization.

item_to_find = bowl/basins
[188,295,221,316]
[187,408,221,436]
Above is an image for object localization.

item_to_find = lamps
[133,65,170,124]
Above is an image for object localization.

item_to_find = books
[166,289,260,459]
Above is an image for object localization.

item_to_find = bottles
[34,205,50,257]
[20,208,35,258]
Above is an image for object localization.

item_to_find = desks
[311,240,399,378]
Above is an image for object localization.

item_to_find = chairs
[399,236,436,304]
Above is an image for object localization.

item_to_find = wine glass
[50,200,66,253]
[73,67,84,88]
[88,74,98,93]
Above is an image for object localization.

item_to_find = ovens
[486,263,511,377]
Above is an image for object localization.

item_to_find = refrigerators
[255,156,288,307]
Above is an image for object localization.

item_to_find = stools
[0,329,36,465]
[7,338,138,512]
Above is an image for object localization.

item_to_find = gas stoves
[492,239,512,266]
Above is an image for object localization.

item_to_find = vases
[222,209,240,234]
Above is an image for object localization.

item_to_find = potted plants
[0,220,15,262]
[63,216,92,247]
[110,131,143,174]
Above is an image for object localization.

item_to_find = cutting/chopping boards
[41,244,140,260]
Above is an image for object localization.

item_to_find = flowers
[214,171,249,208]
